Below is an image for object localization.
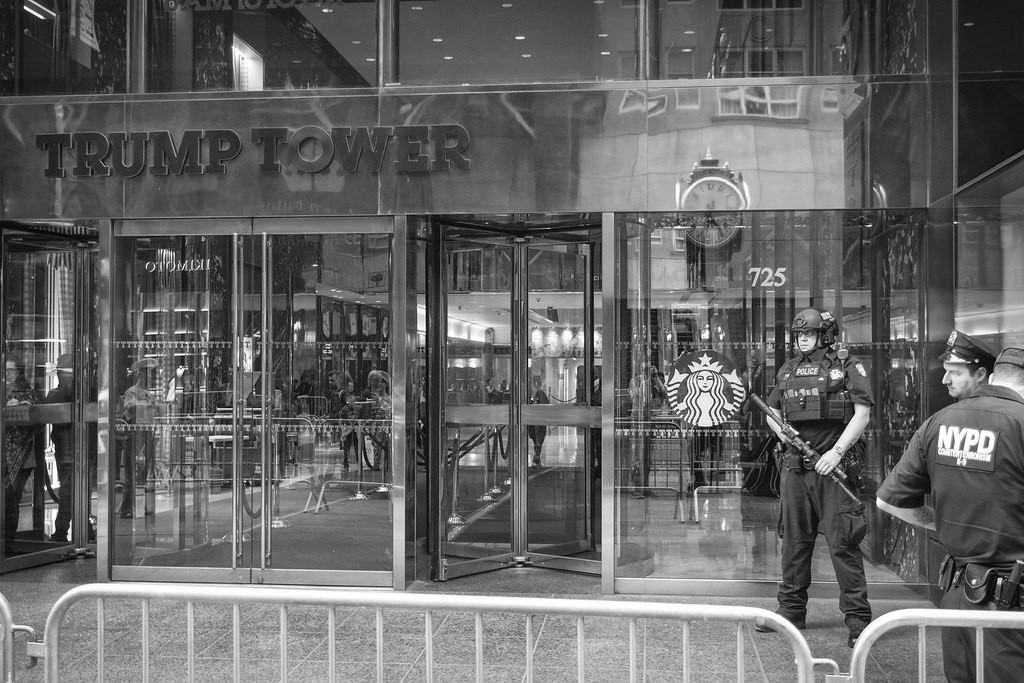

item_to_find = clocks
[681,179,743,246]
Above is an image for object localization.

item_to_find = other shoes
[645,490,660,497]
[48,531,68,542]
[121,513,133,519]
[532,454,544,470]
[342,465,349,472]
[144,511,153,515]
[630,490,644,499]
[687,482,708,491]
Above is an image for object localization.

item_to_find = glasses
[5,368,18,374]
[796,330,814,337]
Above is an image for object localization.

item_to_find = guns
[751,392,863,507]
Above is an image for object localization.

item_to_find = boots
[752,544,782,577]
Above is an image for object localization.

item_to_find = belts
[959,574,966,586]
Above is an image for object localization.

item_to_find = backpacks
[528,399,540,444]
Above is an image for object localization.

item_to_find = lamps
[532,325,598,358]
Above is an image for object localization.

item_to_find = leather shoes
[848,617,872,648]
[754,609,807,632]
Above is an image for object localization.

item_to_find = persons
[624,351,725,500]
[417,367,551,471]
[117,350,391,519]
[878,348,1024,683]
[756,307,875,644]
[44,355,96,544]
[4,353,42,554]
[937,328,999,401]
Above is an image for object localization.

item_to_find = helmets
[791,309,839,356]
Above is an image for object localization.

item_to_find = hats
[130,358,159,372]
[937,329,997,368]
[994,346,1024,366]
